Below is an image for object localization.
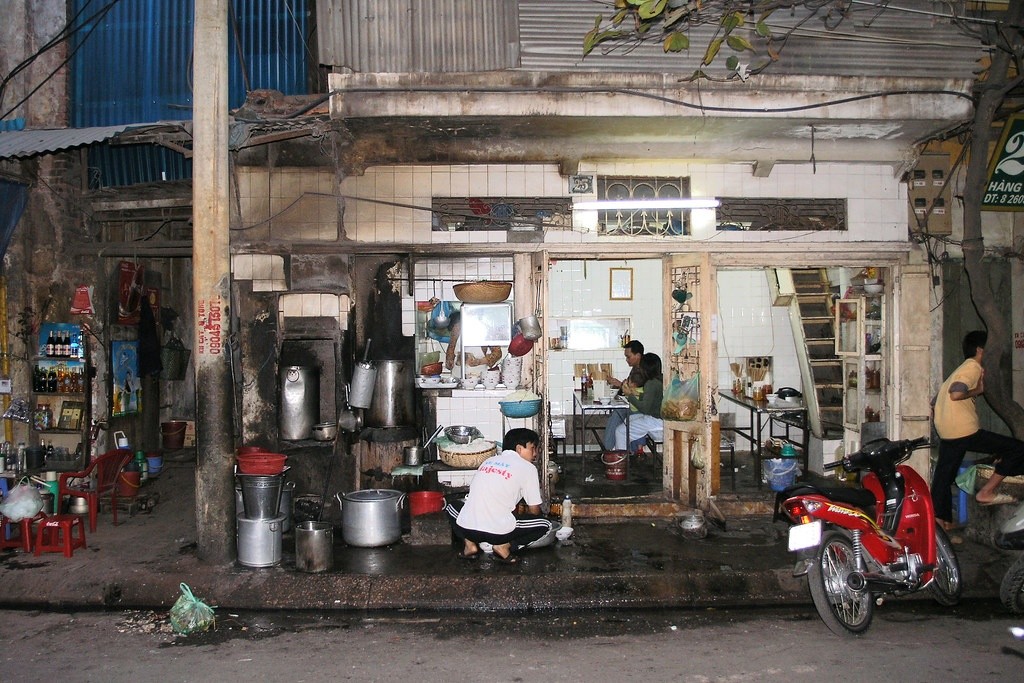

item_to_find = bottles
[730,375,772,400]
[1,435,80,473]
[561,494,571,529]
[30,326,86,392]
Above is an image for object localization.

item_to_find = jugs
[113,430,132,458]
[779,443,799,487]
[31,470,59,515]
[134,449,148,480]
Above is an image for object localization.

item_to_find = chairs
[58,448,135,534]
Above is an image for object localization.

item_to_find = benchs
[645,430,663,484]
[719,432,735,491]
[547,416,567,480]
[768,414,808,463]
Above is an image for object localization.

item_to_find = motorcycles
[777,436,963,637]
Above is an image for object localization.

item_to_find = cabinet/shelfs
[834,293,899,461]
[26,335,91,473]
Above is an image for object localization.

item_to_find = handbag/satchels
[659,369,700,421]
[0,475,44,520]
[170,581,215,634]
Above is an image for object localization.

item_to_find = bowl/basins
[609,387,620,398]
[554,525,573,540]
[478,542,494,553]
[863,283,882,294]
[444,425,480,444]
[525,519,563,547]
[457,350,521,389]
[764,393,778,402]
[598,396,612,406]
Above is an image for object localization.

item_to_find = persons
[930,331,1024,543]
[593,341,646,461]
[122,372,132,412]
[623,372,644,411]
[444,323,503,385]
[611,353,664,452]
[454,427,552,565]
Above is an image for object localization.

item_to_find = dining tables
[0,466,47,489]
[573,388,630,479]
[718,387,809,488]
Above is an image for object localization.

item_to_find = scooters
[995,498,1024,617]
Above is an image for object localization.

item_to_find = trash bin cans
[601,449,629,481]
[762,459,798,492]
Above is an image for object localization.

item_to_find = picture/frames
[609,268,634,301]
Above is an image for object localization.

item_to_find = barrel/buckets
[235,467,285,519]
[145,452,164,472]
[118,469,141,496]
[159,418,187,448]
[407,488,444,518]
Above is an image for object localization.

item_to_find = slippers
[488,552,519,565]
[456,551,481,559]
[978,493,1019,506]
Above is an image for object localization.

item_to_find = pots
[311,420,338,441]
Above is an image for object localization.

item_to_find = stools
[34,514,87,558]
[0,513,49,554]
